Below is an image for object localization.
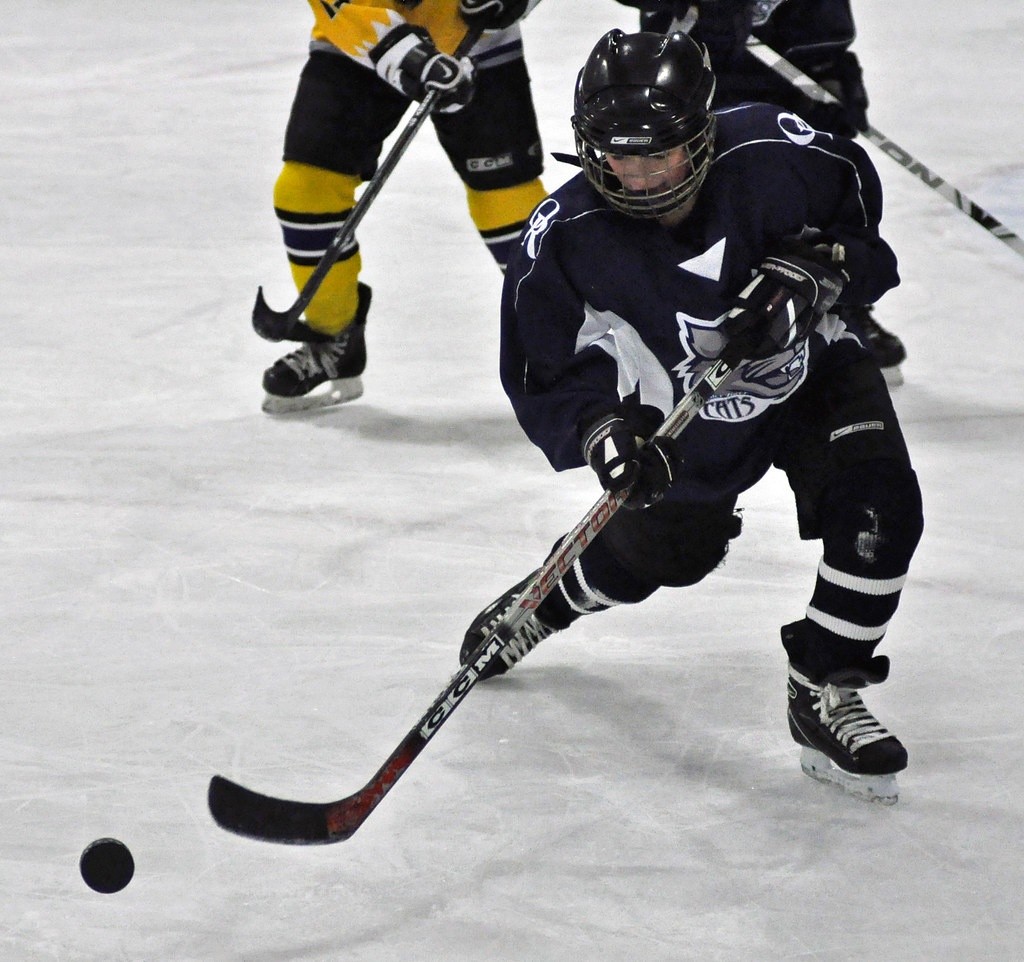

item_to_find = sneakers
[829,303,907,387]
[261,282,371,414]
[781,619,908,806]
[461,533,621,677]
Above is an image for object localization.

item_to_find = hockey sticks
[207,309,783,847]
[250,0,505,341]
[745,33,1024,260]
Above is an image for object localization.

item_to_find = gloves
[459,0,541,34]
[717,253,843,361]
[580,411,685,511]
[368,23,479,114]
[790,52,869,138]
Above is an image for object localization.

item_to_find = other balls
[78,837,135,894]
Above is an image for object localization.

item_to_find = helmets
[551,29,718,220]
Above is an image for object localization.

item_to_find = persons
[460,27,923,807]
[615,0,908,387]
[248,1,548,415]
